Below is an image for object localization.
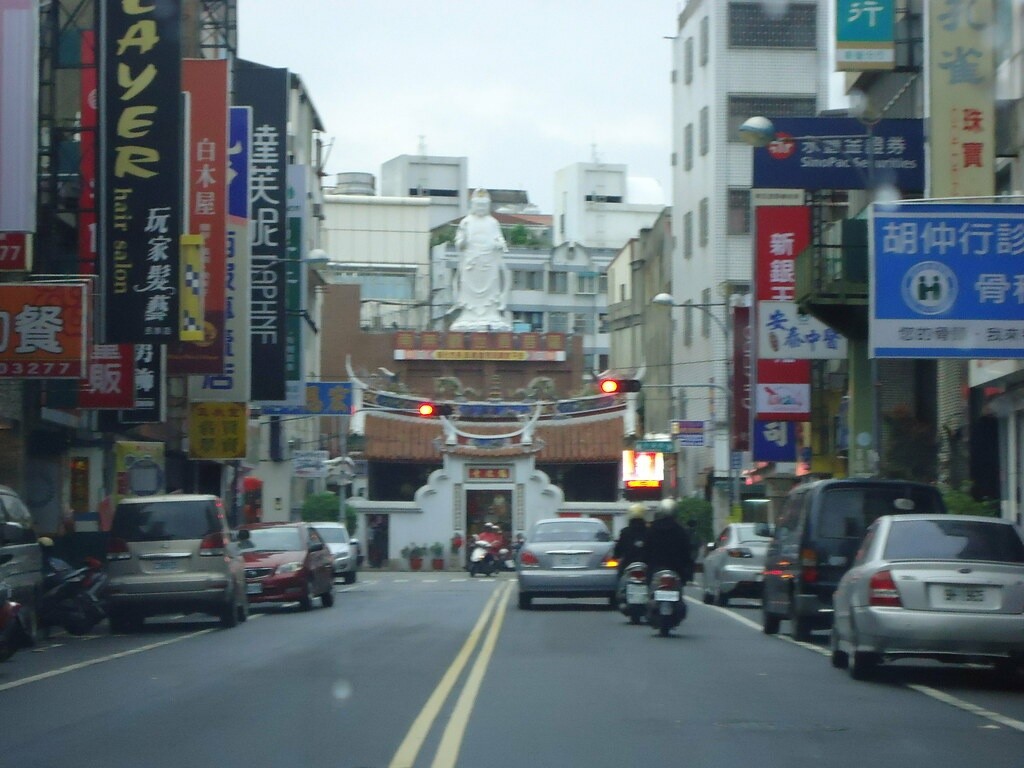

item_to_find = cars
[106,496,252,632]
[232,520,339,610]
[701,522,775,606]
[309,520,361,584]
[0,486,44,603]
[514,518,621,610]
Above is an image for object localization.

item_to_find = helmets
[491,525,500,530]
[627,504,646,521]
[654,499,680,520]
[484,523,493,528]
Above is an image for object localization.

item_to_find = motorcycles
[632,540,699,639]
[595,530,653,625]
[467,533,499,579]
[34,536,108,634]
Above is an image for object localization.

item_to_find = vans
[832,513,1024,694]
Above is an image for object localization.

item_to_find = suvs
[763,478,948,641]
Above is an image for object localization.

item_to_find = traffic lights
[419,403,452,417]
[599,379,642,393]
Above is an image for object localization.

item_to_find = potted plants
[400,542,429,570]
[430,541,445,570]
[675,496,716,572]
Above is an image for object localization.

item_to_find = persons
[449,188,513,332]
[480,522,502,574]
[647,499,693,588]
[614,503,650,576]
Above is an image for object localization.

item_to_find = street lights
[741,114,885,478]
[653,294,730,480]
